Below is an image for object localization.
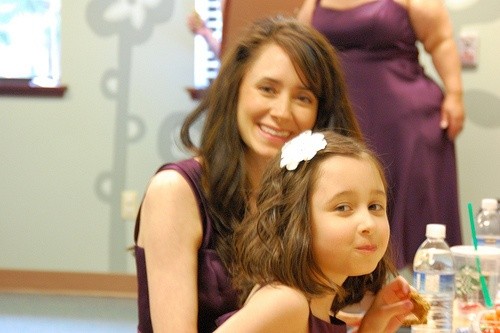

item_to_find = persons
[189,0,465,271]
[211,129,414,333]
[134,17,362,333]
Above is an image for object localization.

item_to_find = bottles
[410,224,455,333]
[475,198,499,246]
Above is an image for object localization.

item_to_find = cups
[450,244,500,333]
